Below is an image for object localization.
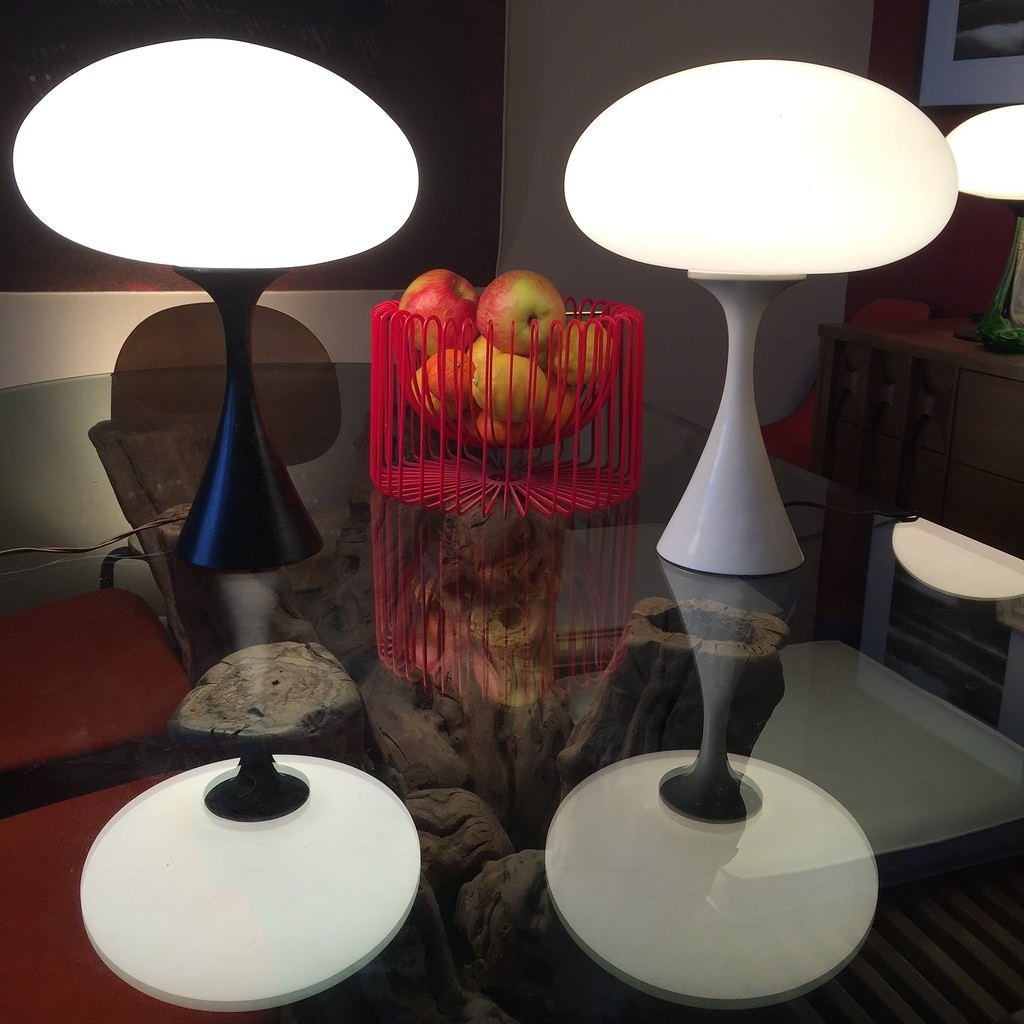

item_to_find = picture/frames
[913,0,1024,115]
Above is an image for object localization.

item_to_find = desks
[0,364,1024,1024]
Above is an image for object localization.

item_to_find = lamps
[13,38,419,568]
[944,105,1024,352]
[564,58,959,575]
[81,575,421,1014]
[543,556,877,1007]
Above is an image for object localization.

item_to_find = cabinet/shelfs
[809,317,1024,562]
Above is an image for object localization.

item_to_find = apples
[401,269,564,365]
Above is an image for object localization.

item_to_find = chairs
[0,588,193,775]
[88,304,342,656]
[760,297,934,471]
[0,769,268,1024]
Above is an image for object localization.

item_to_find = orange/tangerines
[412,320,612,446]
